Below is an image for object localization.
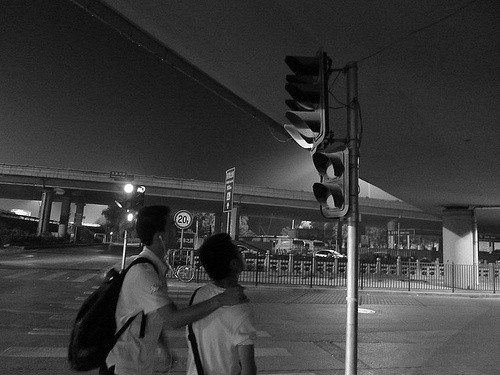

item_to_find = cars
[315,249,347,259]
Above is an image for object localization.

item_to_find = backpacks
[68,257,159,372]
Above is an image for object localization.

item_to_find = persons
[185,232,258,375]
[99,205,250,375]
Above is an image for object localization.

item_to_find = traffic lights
[311,144,349,218]
[280,45,326,155]
[121,180,136,202]
[123,208,135,230]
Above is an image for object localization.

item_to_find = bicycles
[165,255,195,283]
